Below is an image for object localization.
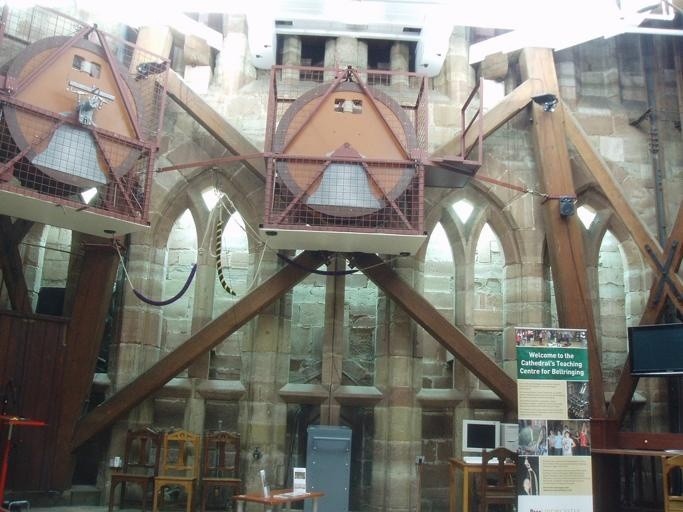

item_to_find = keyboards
[463,456,506,464]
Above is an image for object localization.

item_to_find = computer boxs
[500,423,519,463]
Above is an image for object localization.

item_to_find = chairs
[473,449,518,512]
[661,454,683,512]
[109,424,241,512]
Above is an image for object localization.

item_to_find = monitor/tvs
[462,420,500,453]
[628,324,683,373]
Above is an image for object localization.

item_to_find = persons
[520,478,531,495]
[516,329,570,348]
[548,425,588,456]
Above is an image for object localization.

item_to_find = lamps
[137,63,166,78]
[530,93,557,112]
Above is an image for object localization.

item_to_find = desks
[449,457,517,512]
[230,489,325,512]
[0,415,48,512]
